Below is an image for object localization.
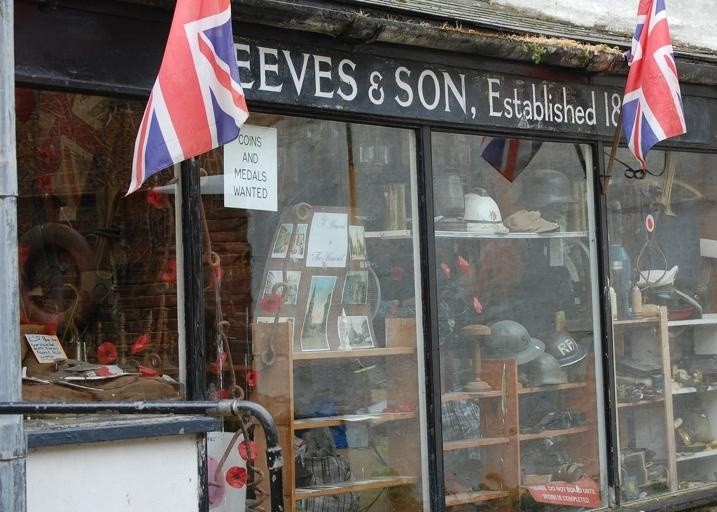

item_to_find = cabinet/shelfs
[429,132,598,512]
[609,295,717,504]
[252,114,422,512]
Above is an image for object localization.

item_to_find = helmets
[481,320,587,385]
[513,170,579,208]
[456,187,503,222]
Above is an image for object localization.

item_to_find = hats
[502,210,560,233]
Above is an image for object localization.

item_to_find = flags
[620,0,689,171]
[121,0,250,198]
[476,136,544,183]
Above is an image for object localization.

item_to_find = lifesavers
[18,224,97,329]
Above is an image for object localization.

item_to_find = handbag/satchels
[297,452,360,512]
[442,399,481,461]
